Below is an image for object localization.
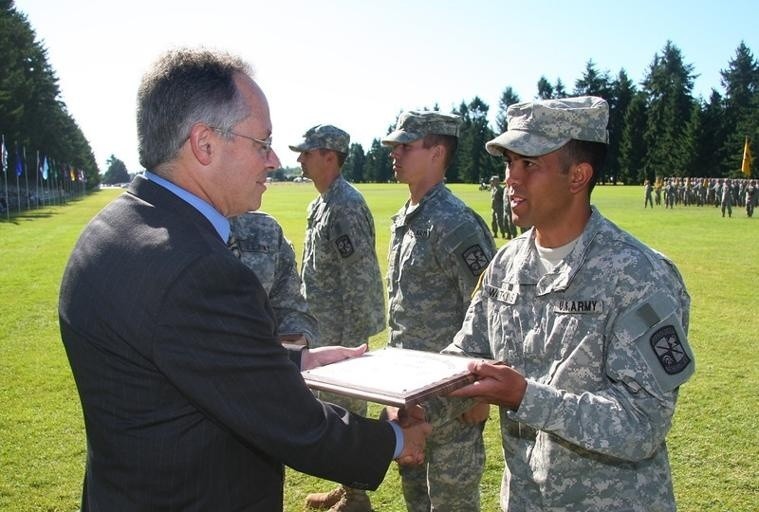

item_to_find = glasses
[179,126,272,160]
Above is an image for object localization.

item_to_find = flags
[1,135,9,172]
[42,155,49,180]
[64,165,88,183]
[742,138,753,176]
[14,143,22,176]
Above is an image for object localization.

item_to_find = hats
[382,110,463,146]
[289,124,350,154]
[485,96,610,157]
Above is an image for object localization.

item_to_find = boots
[306,484,371,512]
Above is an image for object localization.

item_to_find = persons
[644,177,759,217]
[503,188,517,239]
[58,47,432,512]
[228,211,320,349]
[489,176,505,238]
[381,110,498,512]
[287,123,387,512]
[397,96,696,512]
